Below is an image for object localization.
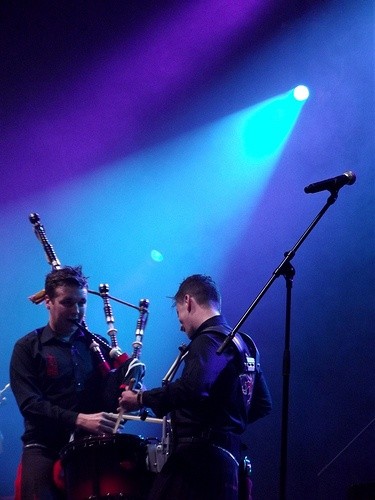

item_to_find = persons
[117,274,272,500]
[8,264,143,500]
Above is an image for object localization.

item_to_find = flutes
[30,212,152,415]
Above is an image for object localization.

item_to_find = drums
[59,433,149,500]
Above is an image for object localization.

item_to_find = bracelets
[136,391,142,410]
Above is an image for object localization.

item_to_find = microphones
[303,170,356,193]
